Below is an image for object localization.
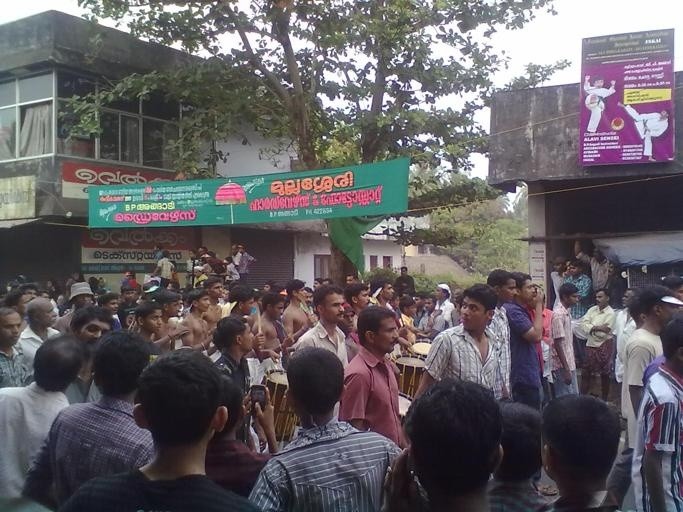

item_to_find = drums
[407,343,432,362]
[394,354,426,399]
[397,390,412,447]
[261,370,297,444]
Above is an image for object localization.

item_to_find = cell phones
[251,385,264,414]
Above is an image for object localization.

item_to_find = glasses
[86,324,107,335]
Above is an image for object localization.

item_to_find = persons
[623,104,667,164]
[2,242,681,512]
[579,74,617,134]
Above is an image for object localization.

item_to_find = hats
[69,282,95,302]
